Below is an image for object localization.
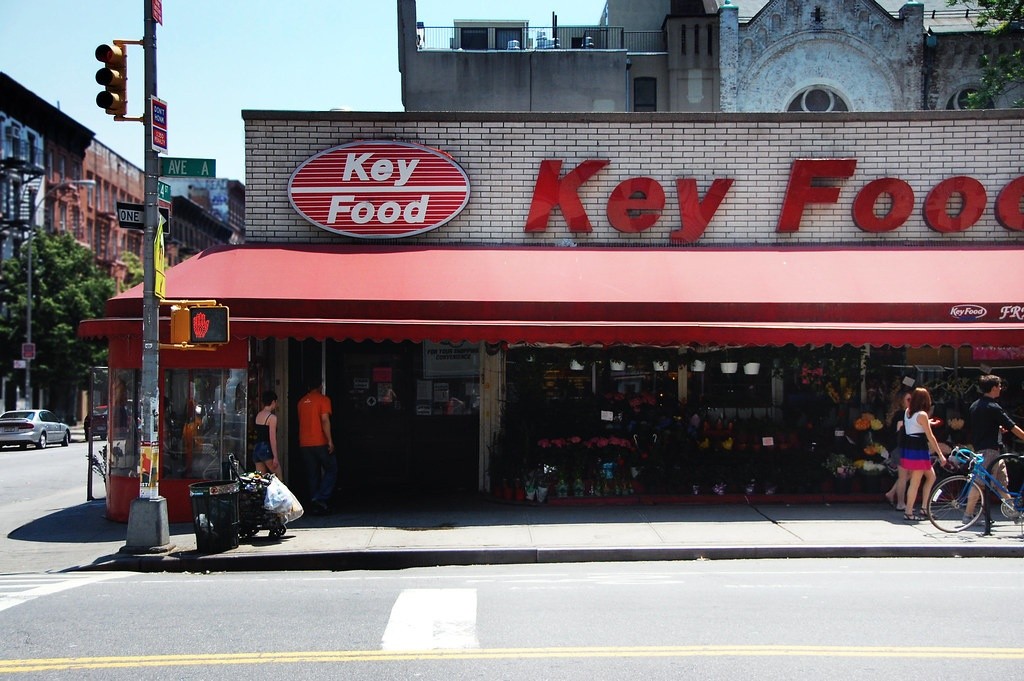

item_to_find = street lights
[25,178,98,409]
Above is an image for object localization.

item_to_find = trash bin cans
[188,481,240,552]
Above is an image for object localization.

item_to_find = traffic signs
[115,204,172,235]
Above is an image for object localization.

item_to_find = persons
[962,375,1024,525]
[253,390,281,481]
[297,376,337,516]
[885,388,947,520]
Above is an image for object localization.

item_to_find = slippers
[897,505,916,511]
[885,493,895,507]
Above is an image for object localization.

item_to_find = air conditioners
[6,126,20,139]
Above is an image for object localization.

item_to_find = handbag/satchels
[264,476,304,524]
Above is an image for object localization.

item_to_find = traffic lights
[188,305,234,344]
[94,43,129,118]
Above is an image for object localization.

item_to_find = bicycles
[926,446,1024,539]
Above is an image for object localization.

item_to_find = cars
[0,409,72,449]
[82,404,144,442]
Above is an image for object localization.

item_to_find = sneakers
[962,513,982,525]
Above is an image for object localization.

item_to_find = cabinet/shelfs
[193,412,246,480]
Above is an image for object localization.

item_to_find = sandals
[919,508,938,518]
[903,514,925,521]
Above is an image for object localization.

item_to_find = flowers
[494,350,972,489]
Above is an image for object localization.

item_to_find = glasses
[906,398,911,402]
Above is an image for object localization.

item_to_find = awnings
[77,245,1024,348]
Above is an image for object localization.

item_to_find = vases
[500,354,963,504]
